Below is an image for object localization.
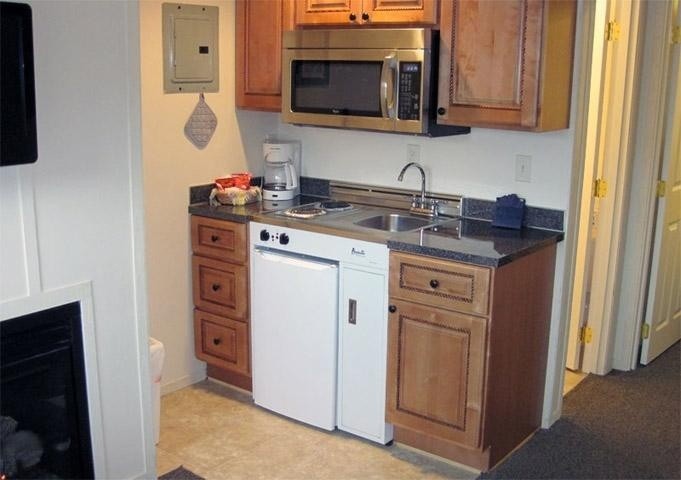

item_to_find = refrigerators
[250,250,337,430]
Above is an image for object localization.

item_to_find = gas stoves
[264,199,366,220]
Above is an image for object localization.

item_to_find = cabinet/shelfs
[438,0,540,130]
[236,0,294,112]
[194,219,249,373]
[388,252,491,450]
[294,0,438,27]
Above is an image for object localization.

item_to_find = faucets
[398,161,426,203]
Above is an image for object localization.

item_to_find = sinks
[352,212,435,236]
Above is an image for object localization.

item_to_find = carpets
[481,340,680,478]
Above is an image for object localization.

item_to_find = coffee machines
[260,139,298,200]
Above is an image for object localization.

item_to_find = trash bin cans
[150,337,165,446]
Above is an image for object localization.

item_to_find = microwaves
[281,29,472,136]
